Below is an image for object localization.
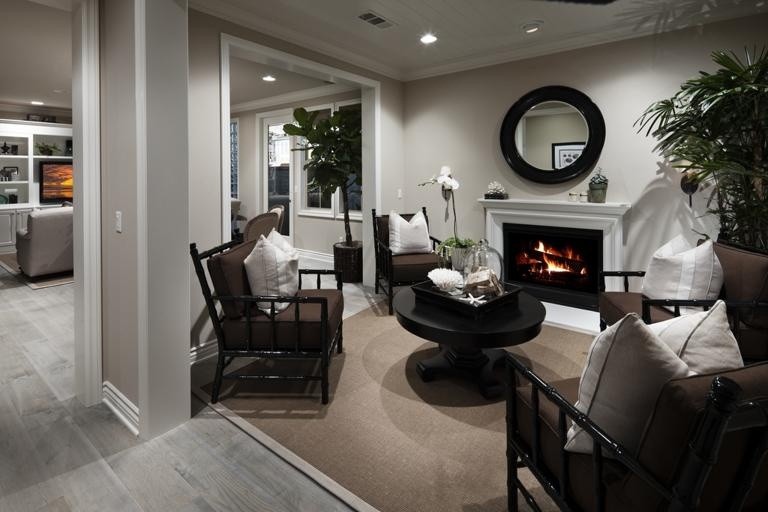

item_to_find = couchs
[16,206,74,279]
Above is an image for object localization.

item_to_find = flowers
[421,166,471,247]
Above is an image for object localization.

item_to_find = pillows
[562,300,745,463]
[243,229,300,314]
[387,212,434,256]
[640,235,723,316]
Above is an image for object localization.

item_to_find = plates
[411,280,523,314]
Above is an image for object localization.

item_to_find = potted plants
[283,107,362,282]
[588,168,608,203]
[632,45,768,251]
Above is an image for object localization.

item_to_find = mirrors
[500,84,607,184]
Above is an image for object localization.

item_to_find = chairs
[504,354,768,512]
[600,241,768,360]
[191,242,343,403]
[371,208,450,316]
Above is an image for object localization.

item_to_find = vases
[452,247,468,273]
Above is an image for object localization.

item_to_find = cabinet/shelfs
[0,120,73,254]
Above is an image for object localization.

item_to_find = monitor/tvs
[39,160,73,204]
